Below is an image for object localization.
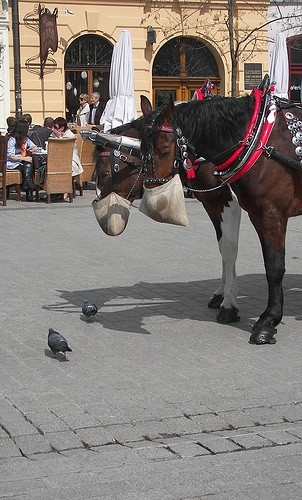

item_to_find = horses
[90,96,243,321]
[139,95,302,344]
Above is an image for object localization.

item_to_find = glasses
[79,98,83,101]
[54,126,60,129]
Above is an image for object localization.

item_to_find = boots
[25,189,39,202]
[22,164,36,189]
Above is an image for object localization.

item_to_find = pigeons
[47,328,72,361]
[81,299,99,324]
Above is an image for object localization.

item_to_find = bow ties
[93,105,96,108]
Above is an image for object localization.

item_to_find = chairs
[0,132,22,206]
[72,134,84,198]
[34,137,77,203]
[73,126,96,187]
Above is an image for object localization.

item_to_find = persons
[75,93,91,126]
[45,117,84,200]
[7,119,37,189]
[89,91,104,125]
[35,117,55,149]
[7,116,16,132]
[22,114,42,131]
